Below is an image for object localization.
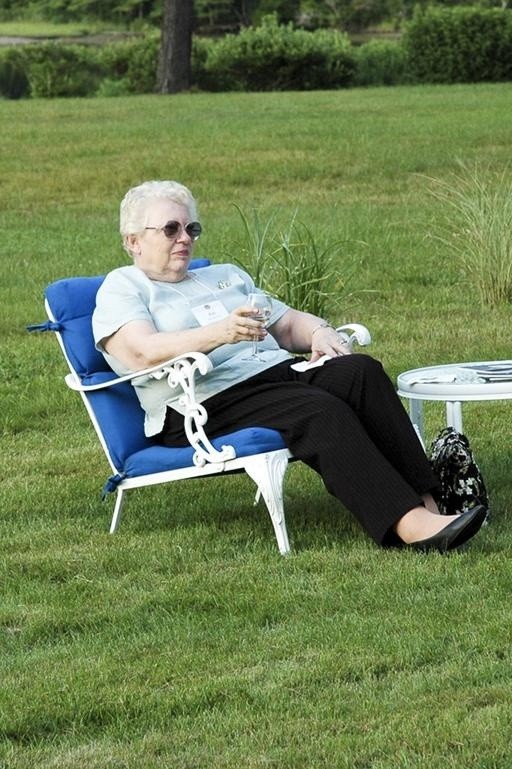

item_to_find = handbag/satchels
[427,426,489,528]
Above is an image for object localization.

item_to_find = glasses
[146,220,202,240]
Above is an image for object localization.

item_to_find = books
[457,362,512,383]
[408,372,457,385]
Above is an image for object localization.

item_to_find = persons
[91,180,486,553]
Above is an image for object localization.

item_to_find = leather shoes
[409,506,488,554]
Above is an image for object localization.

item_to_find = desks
[397,360,512,525]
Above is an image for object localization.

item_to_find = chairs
[26,258,373,557]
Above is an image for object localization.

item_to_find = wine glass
[239,292,273,362]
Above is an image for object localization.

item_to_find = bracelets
[312,323,336,334]
